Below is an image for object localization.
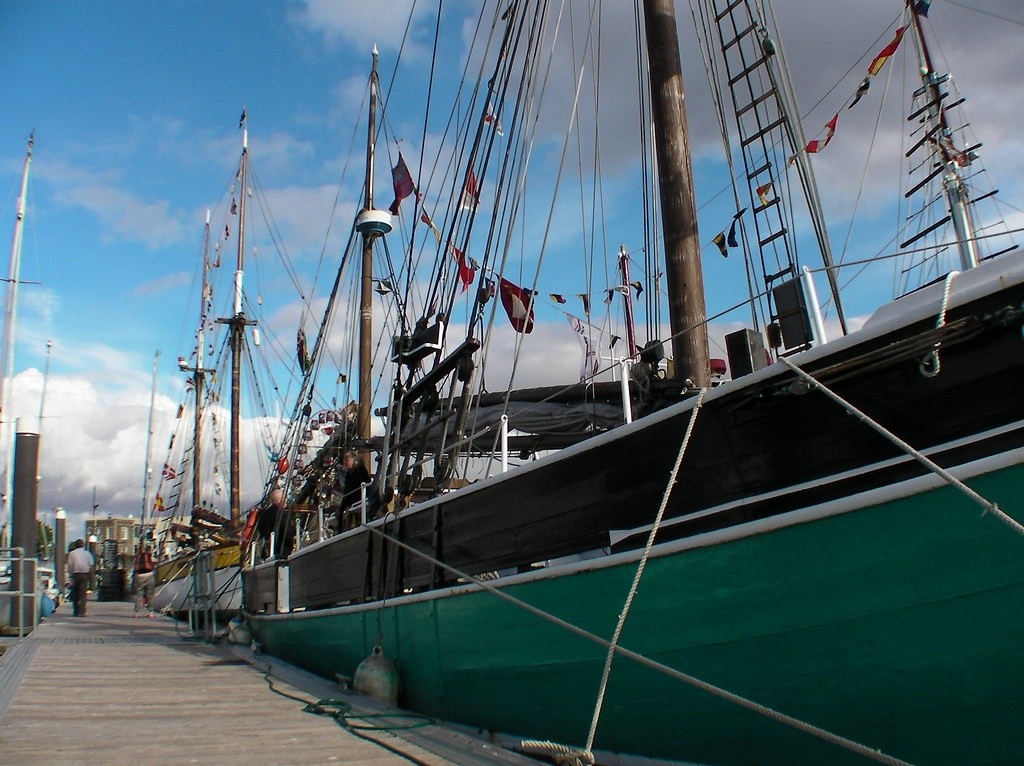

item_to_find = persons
[132,546,156,619]
[68,539,94,617]
[175,534,191,553]
[258,489,297,560]
[336,453,372,534]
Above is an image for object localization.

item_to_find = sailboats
[137,0,1024,766]
[0,125,58,630]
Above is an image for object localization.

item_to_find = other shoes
[147,611,154,618]
[73,611,87,617]
[133,612,140,618]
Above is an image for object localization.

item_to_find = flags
[712,22,913,258]
[390,99,664,382]
[150,199,309,514]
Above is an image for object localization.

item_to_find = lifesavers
[240,509,259,552]
